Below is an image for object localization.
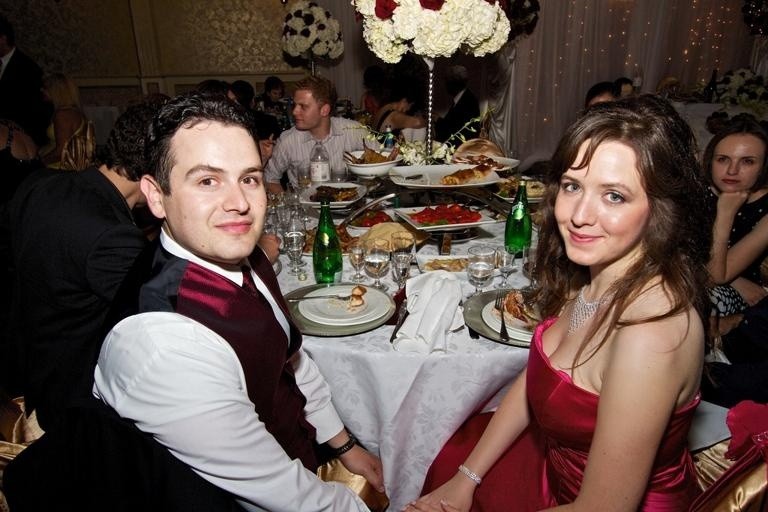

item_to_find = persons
[0,90,180,438]
[88,88,389,511]
[698,115,768,320]
[0,13,54,205]
[614,76,633,98]
[191,55,485,210]
[581,77,618,109]
[396,90,716,512]
[38,67,88,173]
[699,295,768,410]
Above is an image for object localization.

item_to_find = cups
[297,163,311,187]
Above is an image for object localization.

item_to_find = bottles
[313,198,343,283]
[504,181,532,255]
[383,125,394,148]
[309,137,330,182]
[704,70,717,103]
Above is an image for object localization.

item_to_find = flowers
[706,63,764,111]
[281,1,347,65]
[339,103,505,167]
[349,1,515,66]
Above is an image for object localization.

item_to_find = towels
[392,268,467,357]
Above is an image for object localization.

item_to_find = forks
[495,291,509,342]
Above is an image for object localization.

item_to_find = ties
[240,265,274,314]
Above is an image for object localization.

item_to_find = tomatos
[361,212,389,227]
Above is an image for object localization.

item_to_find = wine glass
[468,243,544,299]
[349,231,415,297]
[263,189,307,277]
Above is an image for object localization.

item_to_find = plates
[441,304,465,333]
[387,164,503,189]
[414,254,467,273]
[492,305,533,335]
[492,192,545,204]
[300,182,399,231]
[298,229,357,258]
[393,205,507,231]
[481,300,535,343]
[490,157,520,172]
[279,281,394,336]
[463,289,540,349]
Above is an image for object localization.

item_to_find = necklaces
[567,283,615,338]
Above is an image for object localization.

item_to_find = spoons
[370,173,423,181]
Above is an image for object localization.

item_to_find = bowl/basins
[345,150,403,179]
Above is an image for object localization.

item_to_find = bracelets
[333,433,358,458]
[457,464,483,487]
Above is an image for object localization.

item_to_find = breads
[441,164,491,185]
